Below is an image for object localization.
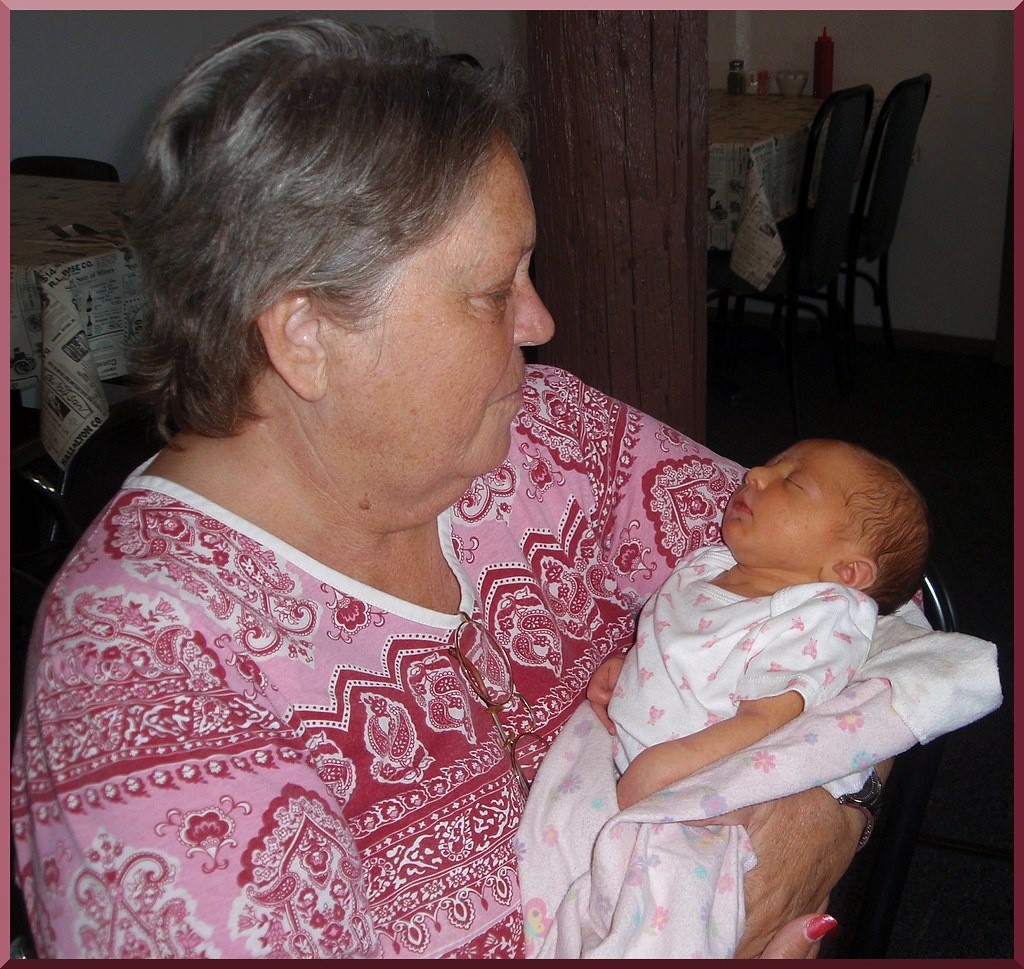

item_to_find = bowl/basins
[776,69,808,96]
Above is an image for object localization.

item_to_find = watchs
[838,766,884,852]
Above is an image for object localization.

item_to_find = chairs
[709,73,933,441]
[843,562,957,958]
[11,155,119,182]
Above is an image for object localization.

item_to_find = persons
[9,19,876,961]
[513,438,929,956]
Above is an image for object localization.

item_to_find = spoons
[72,223,125,240]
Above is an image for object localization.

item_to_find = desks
[10,174,156,543]
[709,88,924,371]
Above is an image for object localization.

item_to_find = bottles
[813,26,834,98]
[727,59,746,95]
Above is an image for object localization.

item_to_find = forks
[47,223,118,245]
